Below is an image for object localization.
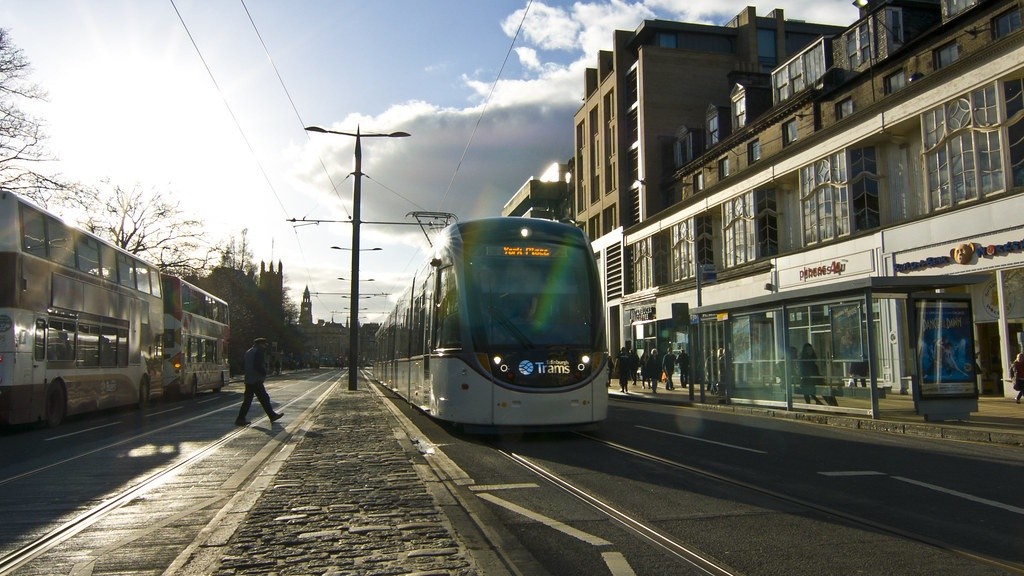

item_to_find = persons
[777,347,796,401]
[704,347,732,395]
[1009,353,1024,404]
[235,338,284,425]
[798,343,824,404]
[850,347,868,387]
[608,347,688,394]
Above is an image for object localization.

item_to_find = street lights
[303,126,411,392]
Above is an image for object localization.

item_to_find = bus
[0,190,166,429]
[161,274,231,399]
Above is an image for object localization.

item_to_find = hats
[253,336,267,342]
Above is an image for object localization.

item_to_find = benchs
[735,383,885,402]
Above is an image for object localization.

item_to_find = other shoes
[625,392,628,394]
[620,389,624,393]
[267,411,284,423]
[681,384,687,388]
[641,385,645,389]
[632,381,636,385]
[670,387,675,390]
[609,384,611,387]
[648,386,651,389]
[235,417,251,425]
[666,389,671,391]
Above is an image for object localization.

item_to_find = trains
[373,217,607,429]
